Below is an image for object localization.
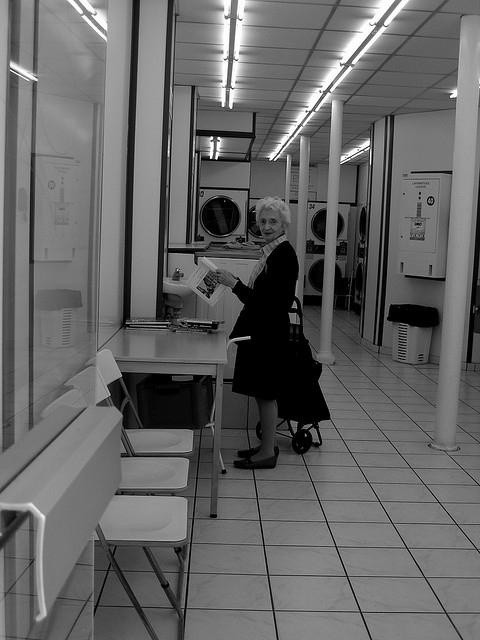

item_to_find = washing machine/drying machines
[248,198,370,317]
[196,159,251,244]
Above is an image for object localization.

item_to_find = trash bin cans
[387,304,440,364]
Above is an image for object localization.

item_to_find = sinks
[163,277,193,297]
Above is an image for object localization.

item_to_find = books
[125,317,225,334]
[187,256,228,306]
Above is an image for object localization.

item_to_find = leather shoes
[234,454,278,470]
[238,446,279,458]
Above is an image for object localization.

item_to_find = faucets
[172,268,185,281]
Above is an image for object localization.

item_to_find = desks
[98,327,227,518]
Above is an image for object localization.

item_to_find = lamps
[269,0,411,162]
[209,136,220,159]
[66,0,108,41]
[9,61,38,82]
[220,0,245,109]
[340,142,370,164]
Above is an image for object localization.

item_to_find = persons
[216,196,299,468]
